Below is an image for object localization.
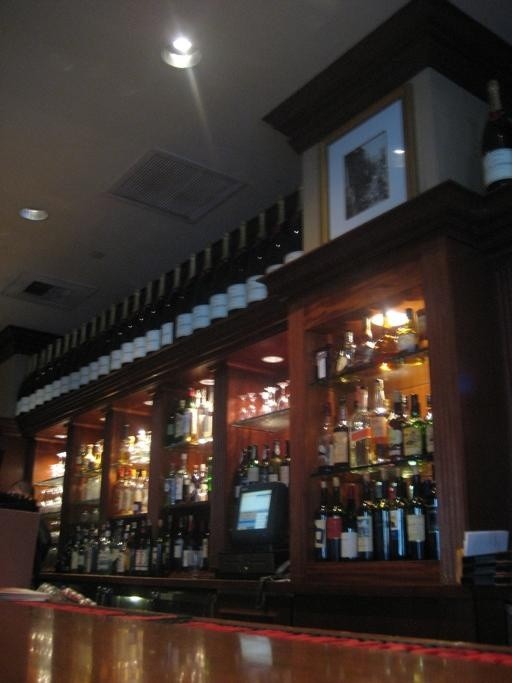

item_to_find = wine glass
[235,376,290,419]
[37,449,67,511]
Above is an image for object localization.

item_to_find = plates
[1,587,52,602]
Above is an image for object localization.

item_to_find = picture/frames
[317,78,422,247]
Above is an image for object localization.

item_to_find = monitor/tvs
[231,482,289,545]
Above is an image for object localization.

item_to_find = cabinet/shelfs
[159,351,230,573]
[24,405,75,577]
[298,262,454,578]
[62,395,112,574]
[113,373,167,574]
[224,312,302,579]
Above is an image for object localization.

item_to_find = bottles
[163,383,214,443]
[326,304,414,376]
[67,515,210,572]
[319,376,430,466]
[162,451,213,506]
[75,427,151,512]
[233,437,291,496]
[14,190,302,419]
[476,74,511,202]
[311,461,440,561]
